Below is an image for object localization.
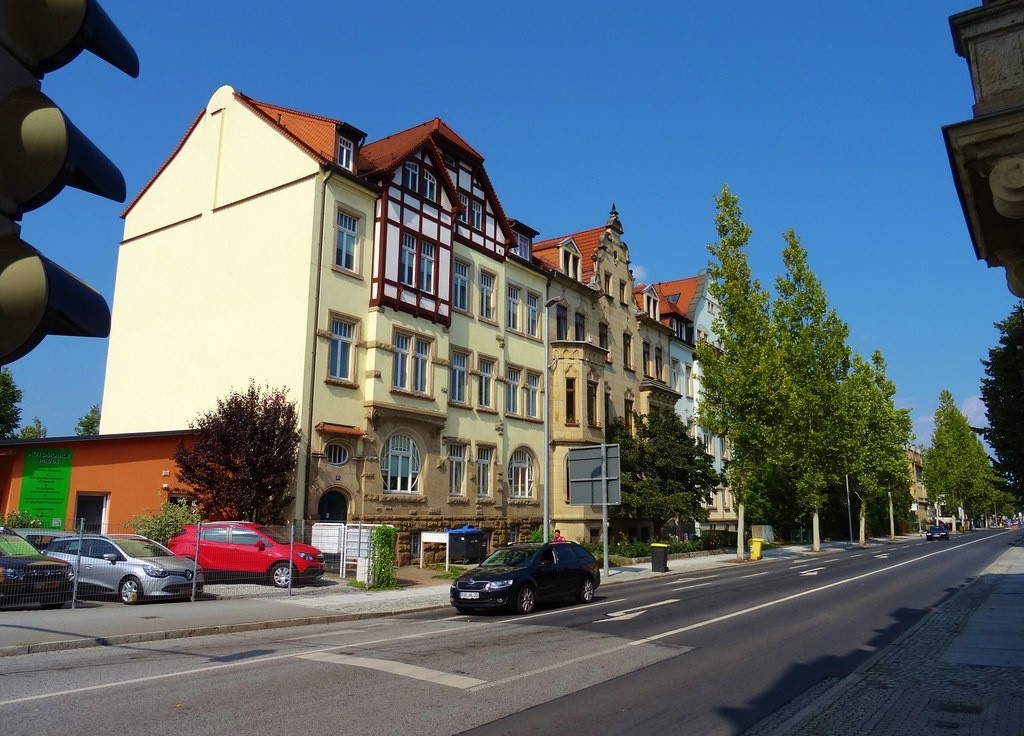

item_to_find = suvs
[0,527,74,609]
[166,520,327,588]
[449,540,602,616]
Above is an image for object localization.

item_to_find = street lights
[544,296,561,541]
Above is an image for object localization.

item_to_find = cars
[926,525,950,541]
[42,534,206,605]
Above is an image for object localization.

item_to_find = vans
[11,525,74,553]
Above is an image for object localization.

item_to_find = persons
[1011,524,1023,541]
[940,522,950,527]
[552,529,566,542]
[609,529,625,546]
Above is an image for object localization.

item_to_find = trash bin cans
[747,538,764,560]
[650,541,669,573]
[445,525,483,565]
